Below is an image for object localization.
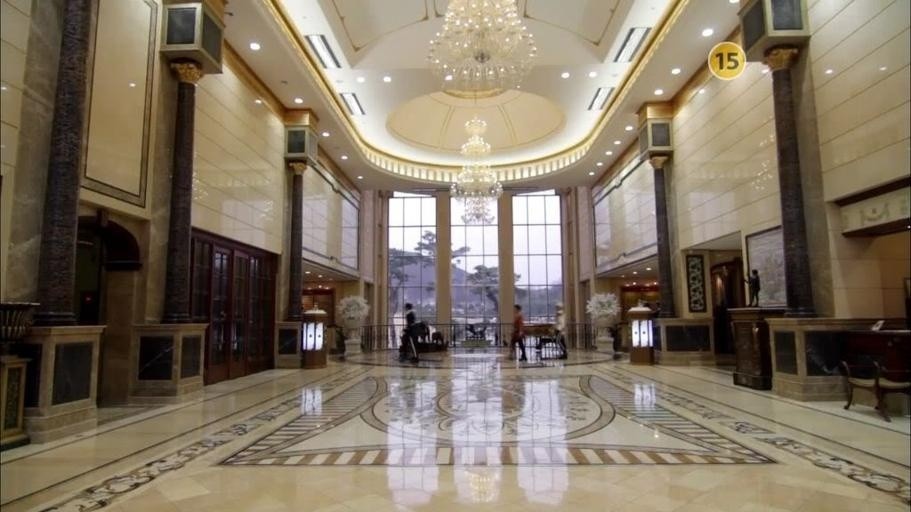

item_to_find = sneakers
[397,355,419,363]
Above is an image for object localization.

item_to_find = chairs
[843,362,911,423]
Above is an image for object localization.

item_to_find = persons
[554,302,568,360]
[399,303,420,364]
[744,270,760,307]
[509,305,527,361]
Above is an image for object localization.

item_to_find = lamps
[428,0,537,80]
[449,90,503,224]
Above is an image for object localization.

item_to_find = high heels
[519,355,527,361]
